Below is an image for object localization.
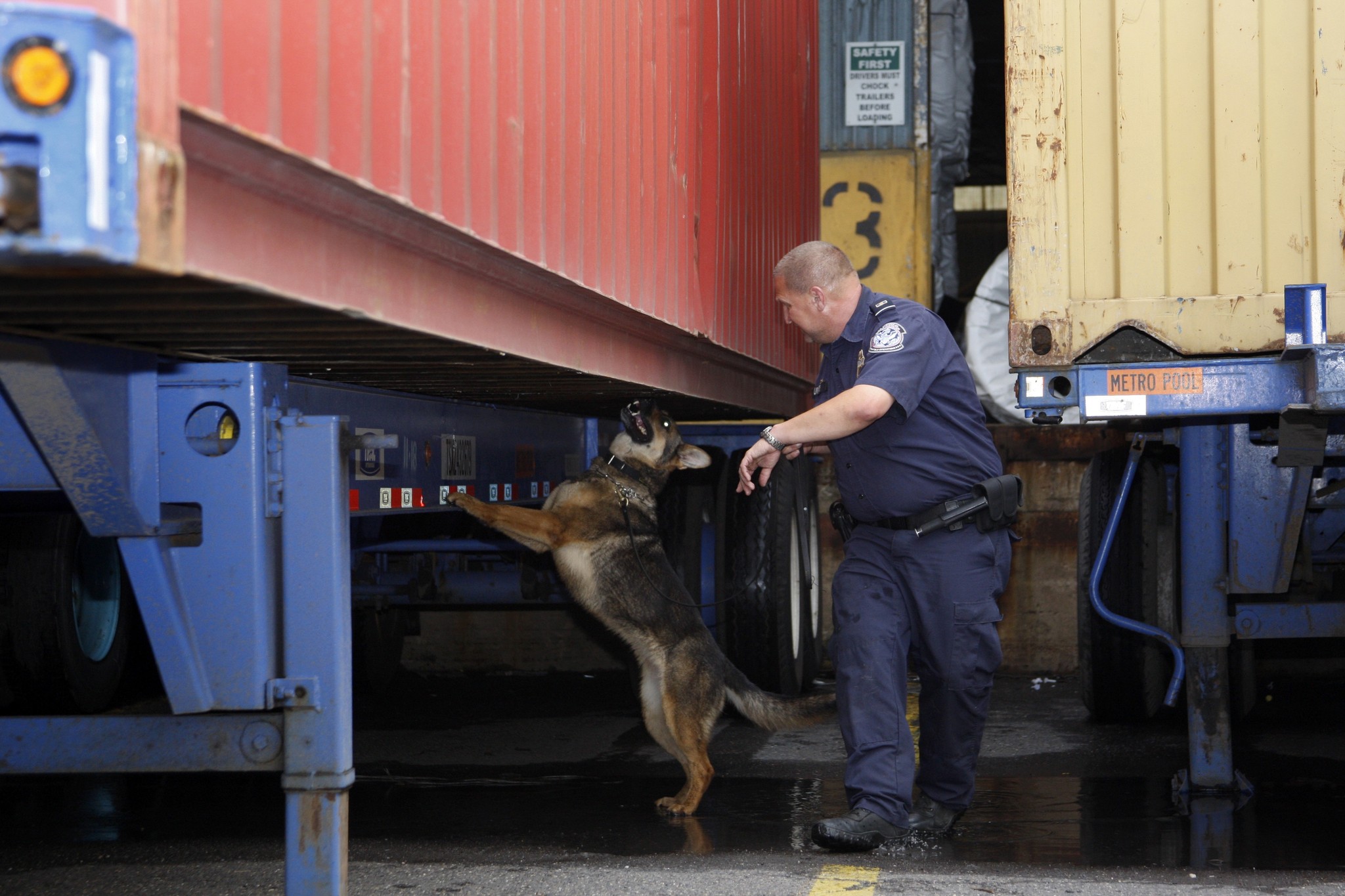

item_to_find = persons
[736,241,1020,853]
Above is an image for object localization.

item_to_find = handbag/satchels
[972,473,1024,534]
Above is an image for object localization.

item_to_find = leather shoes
[908,792,968,835]
[810,800,913,851]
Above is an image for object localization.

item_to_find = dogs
[441,391,845,821]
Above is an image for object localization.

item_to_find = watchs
[758,425,785,452]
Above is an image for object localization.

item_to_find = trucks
[0,4,1344,896]
